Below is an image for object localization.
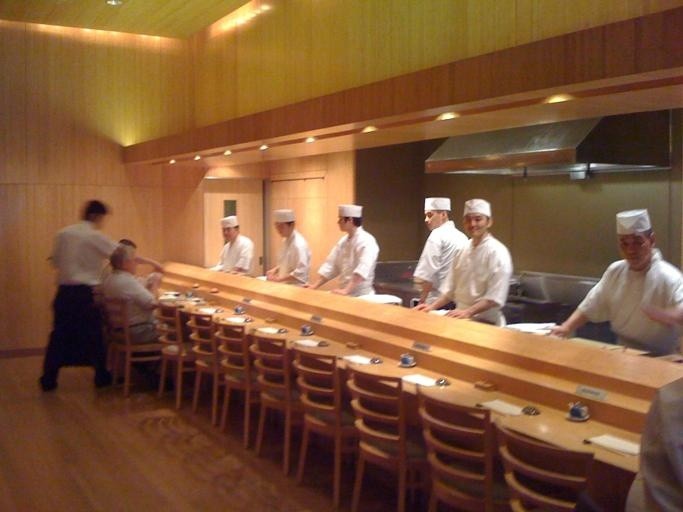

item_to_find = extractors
[423,110,673,178]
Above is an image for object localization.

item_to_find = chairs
[345,361,426,512]
[249,328,305,476]
[416,384,508,512]
[495,418,594,512]
[152,294,195,411]
[187,308,221,423]
[102,294,162,394]
[212,318,259,450]
[291,346,358,508]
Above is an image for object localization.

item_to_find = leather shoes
[39,375,56,391]
[94,372,125,387]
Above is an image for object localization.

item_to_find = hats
[274,208,295,223]
[462,197,492,220]
[424,196,451,212]
[338,204,363,218]
[221,215,239,228]
[615,209,652,235]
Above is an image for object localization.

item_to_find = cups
[300,323,316,336]
[184,289,193,298]
[233,304,245,315]
[399,352,416,368]
[567,402,592,422]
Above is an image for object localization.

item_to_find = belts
[59,283,94,290]
[112,320,151,332]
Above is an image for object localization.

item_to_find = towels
[587,432,642,458]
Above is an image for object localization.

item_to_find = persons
[302,204,380,298]
[37,200,166,394]
[420,198,514,327]
[208,214,257,278]
[413,197,468,311]
[624,376,682,511]
[263,208,312,285]
[539,207,683,356]
